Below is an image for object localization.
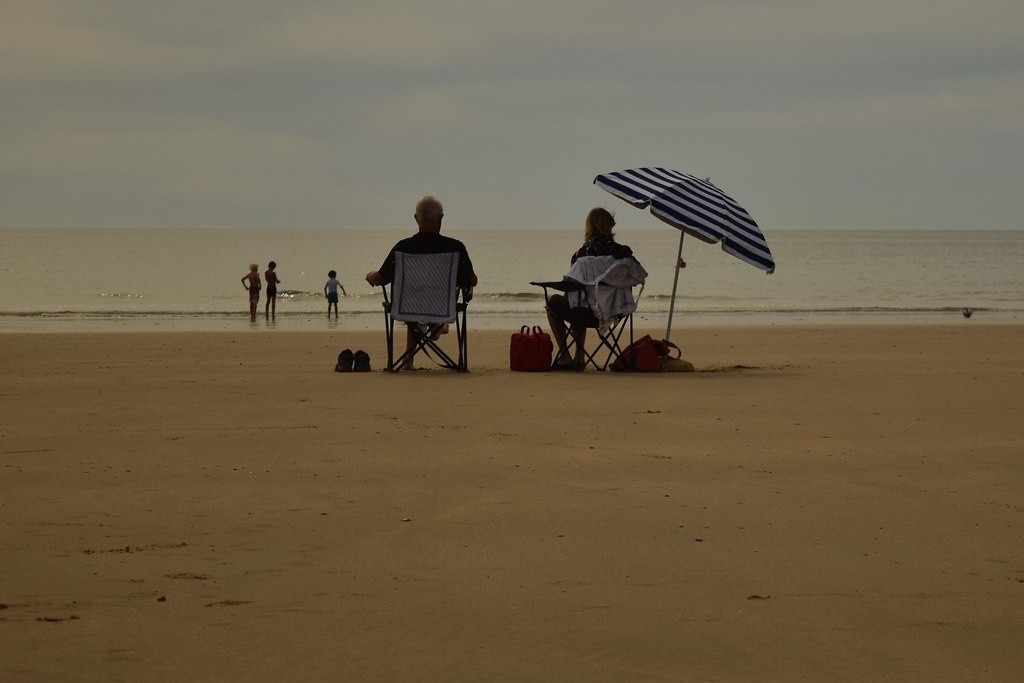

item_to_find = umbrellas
[592,166,776,341]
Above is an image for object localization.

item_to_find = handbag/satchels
[510,324,552,372]
[609,334,683,373]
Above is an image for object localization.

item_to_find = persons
[546,208,634,370]
[241,264,261,314]
[324,270,346,319]
[265,262,280,320]
[366,197,477,370]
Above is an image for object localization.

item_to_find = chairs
[364,250,473,375]
[529,255,648,373]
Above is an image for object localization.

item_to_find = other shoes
[335,348,354,371]
[353,349,371,372]
[574,356,586,371]
[556,355,573,370]
